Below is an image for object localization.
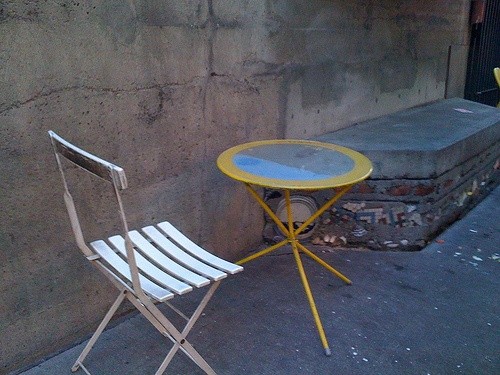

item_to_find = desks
[216,137,374,358]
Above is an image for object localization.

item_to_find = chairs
[48,129,246,375]
